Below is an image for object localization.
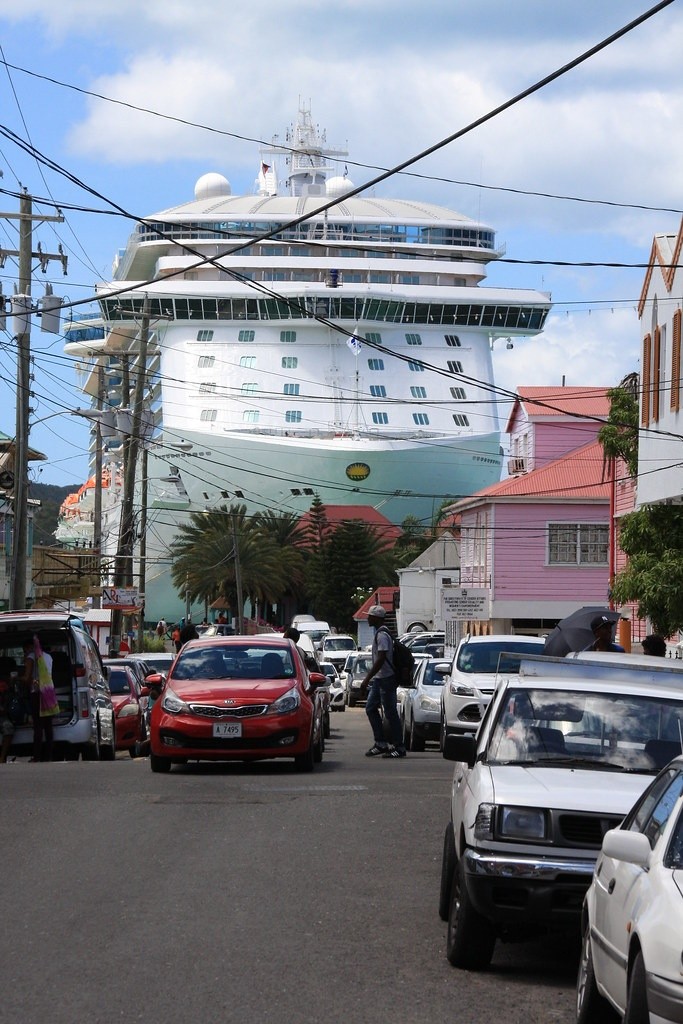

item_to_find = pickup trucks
[437,676,683,971]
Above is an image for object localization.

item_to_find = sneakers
[365,743,389,756]
[382,747,407,758]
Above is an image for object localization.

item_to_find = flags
[343,164,348,178]
[261,163,271,174]
[346,326,362,355]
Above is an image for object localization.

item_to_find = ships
[58,103,555,626]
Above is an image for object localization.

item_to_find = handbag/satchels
[28,679,39,694]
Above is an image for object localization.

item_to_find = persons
[584,615,625,653]
[641,634,667,658]
[283,627,299,643]
[0,679,14,763]
[156,618,167,638]
[181,619,186,631]
[171,627,182,653]
[199,612,228,626]
[360,606,406,758]
[11,632,60,762]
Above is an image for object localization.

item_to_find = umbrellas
[544,606,621,657]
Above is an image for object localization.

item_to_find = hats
[362,605,386,617]
[590,616,616,632]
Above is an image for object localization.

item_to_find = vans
[1,608,116,763]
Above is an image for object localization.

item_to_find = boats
[52,517,94,543]
[76,468,122,512]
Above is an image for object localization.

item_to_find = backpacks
[374,627,415,687]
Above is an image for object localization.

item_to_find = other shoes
[28,756,49,762]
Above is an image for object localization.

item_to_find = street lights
[109,438,194,660]
[8,407,103,613]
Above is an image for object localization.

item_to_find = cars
[98,611,681,777]
[575,751,683,1024]
[143,635,328,773]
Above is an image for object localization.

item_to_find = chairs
[261,653,285,678]
[644,740,682,770]
[528,726,566,754]
[109,672,128,694]
[471,647,490,669]
[199,660,227,676]
